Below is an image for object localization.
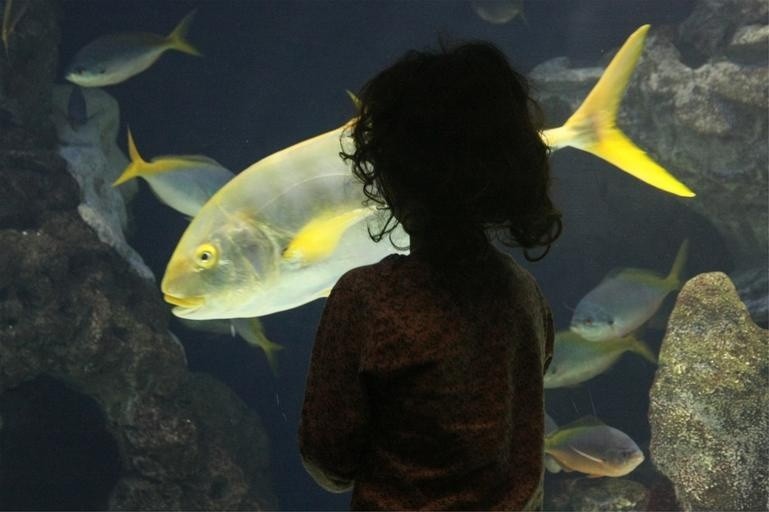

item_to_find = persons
[297,35,561,512]
